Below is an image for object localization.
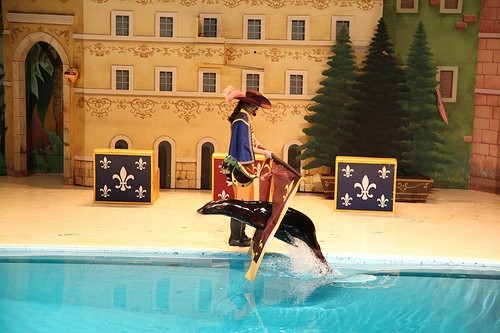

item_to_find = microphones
[243,105,256,116]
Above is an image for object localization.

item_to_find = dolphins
[198,199,333,272]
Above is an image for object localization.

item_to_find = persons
[221,91,276,247]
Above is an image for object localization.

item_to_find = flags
[244,151,306,280]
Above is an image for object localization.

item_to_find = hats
[233,90,272,109]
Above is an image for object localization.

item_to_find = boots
[229,218,251,247]
